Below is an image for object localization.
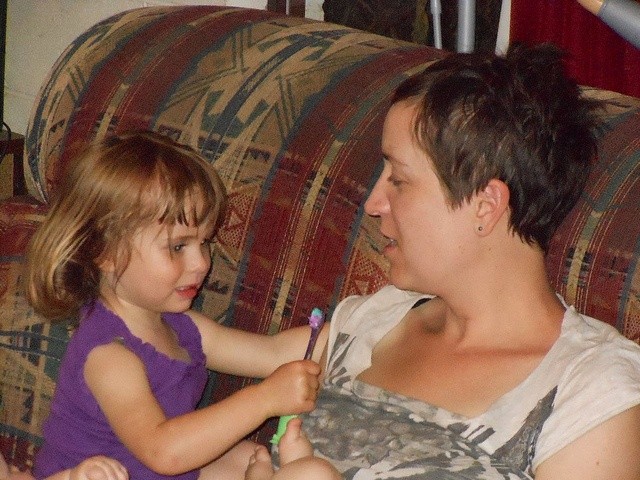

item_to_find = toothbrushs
[272,307,327,452]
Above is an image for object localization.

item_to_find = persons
[275,44,640,480]
[25,127,335,479]
[246,417,339,478]
[0,438,129,480]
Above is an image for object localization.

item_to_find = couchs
[1,1,640,480]
